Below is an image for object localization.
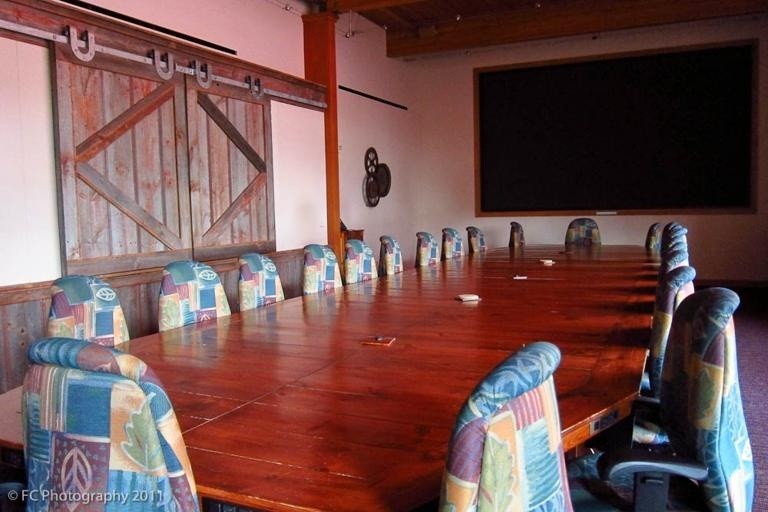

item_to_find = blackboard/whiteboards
[474,36,758,217]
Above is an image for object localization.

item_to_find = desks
[1,234,660,512]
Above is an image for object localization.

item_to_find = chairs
[640,221,699,390]
[301,222,526,296]
[443,340,576,508]
[49,248,285,341]
[566,214,603,243]
[605,285,758,511]
[14,339,205,512]
